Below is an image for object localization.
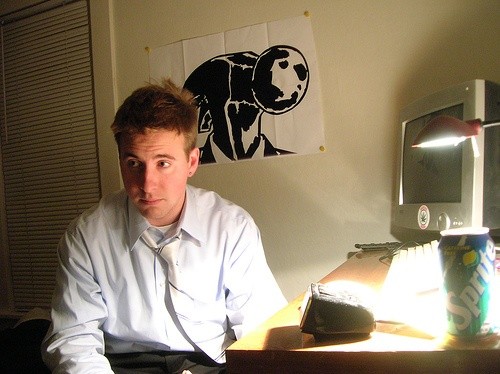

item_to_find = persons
[40,77,289,374]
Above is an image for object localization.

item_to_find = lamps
[410,115,500,149]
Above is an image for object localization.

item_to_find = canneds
[437,226,498,341]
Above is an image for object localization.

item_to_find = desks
[224,238,500,374]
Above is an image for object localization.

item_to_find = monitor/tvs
[390,79,500,243]
